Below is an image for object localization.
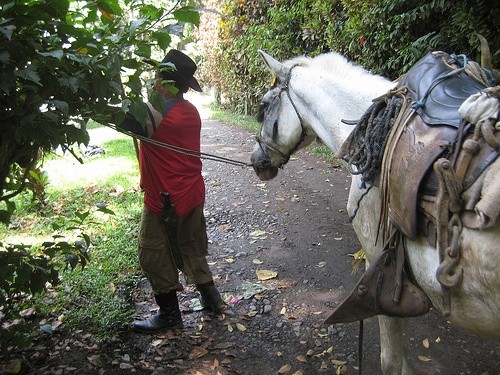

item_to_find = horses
[249,50,500,374]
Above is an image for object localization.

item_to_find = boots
[134,290,185,334]
[198,284,225,315]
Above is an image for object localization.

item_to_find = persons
[92,50,224,333]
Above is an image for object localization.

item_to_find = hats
[142,49,203,92]
